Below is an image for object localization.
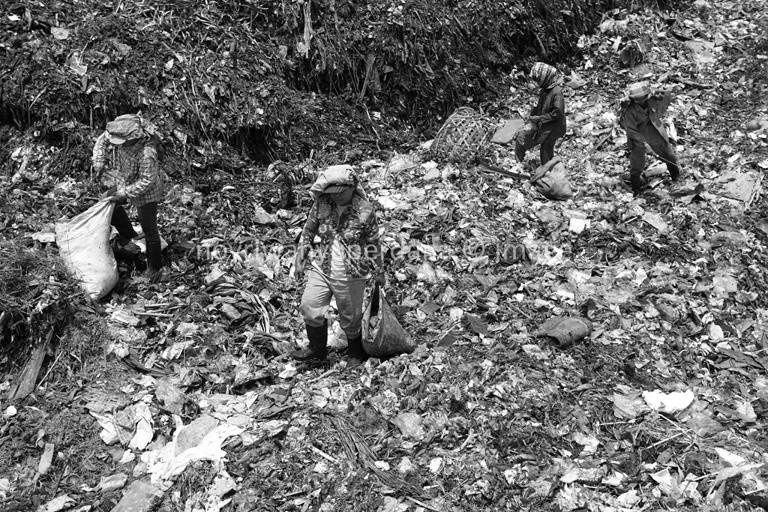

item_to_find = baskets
[430,107,497,164]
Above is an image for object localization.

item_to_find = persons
[515,62,567,166]
[91,114,164,277]
[294,164,385,363]
[619,80,681,194]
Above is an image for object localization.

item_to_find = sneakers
[144,242,163,272]
[110,227,138,247]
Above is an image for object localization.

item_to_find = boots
[346,327,367,364]
[290,317,328,361]
